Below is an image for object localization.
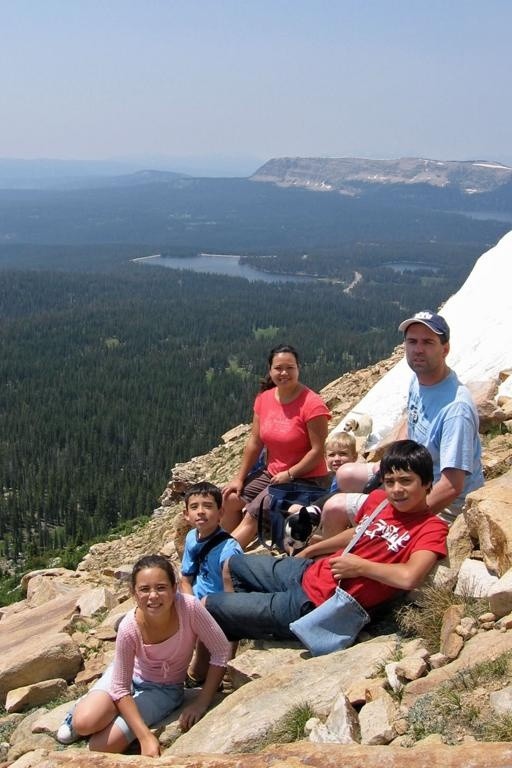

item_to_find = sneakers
[57,710,80,744]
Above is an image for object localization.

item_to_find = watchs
[287,469,295,481]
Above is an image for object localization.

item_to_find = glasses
[137,586,167,592]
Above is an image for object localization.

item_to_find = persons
[185,442,449,691]
[320,310,485,537]
[178,481,245,598]
[57,555,232,758]
[220,344,332,549]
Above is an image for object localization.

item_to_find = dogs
[277,487,342,558]
[343,409,373,449]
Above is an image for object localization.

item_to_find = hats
[398,310,450,335]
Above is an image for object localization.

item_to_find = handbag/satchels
[289,587,370,657]
